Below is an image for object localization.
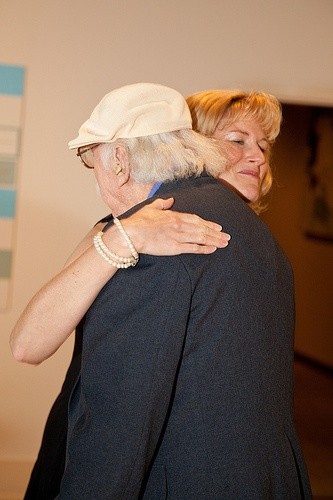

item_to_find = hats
[67,83,191,150]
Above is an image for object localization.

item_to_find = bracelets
[93,217,138,268]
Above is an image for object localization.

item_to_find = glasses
[75,143,104,169]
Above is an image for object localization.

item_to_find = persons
[23,83,312,500]
[8,90,281,500]
